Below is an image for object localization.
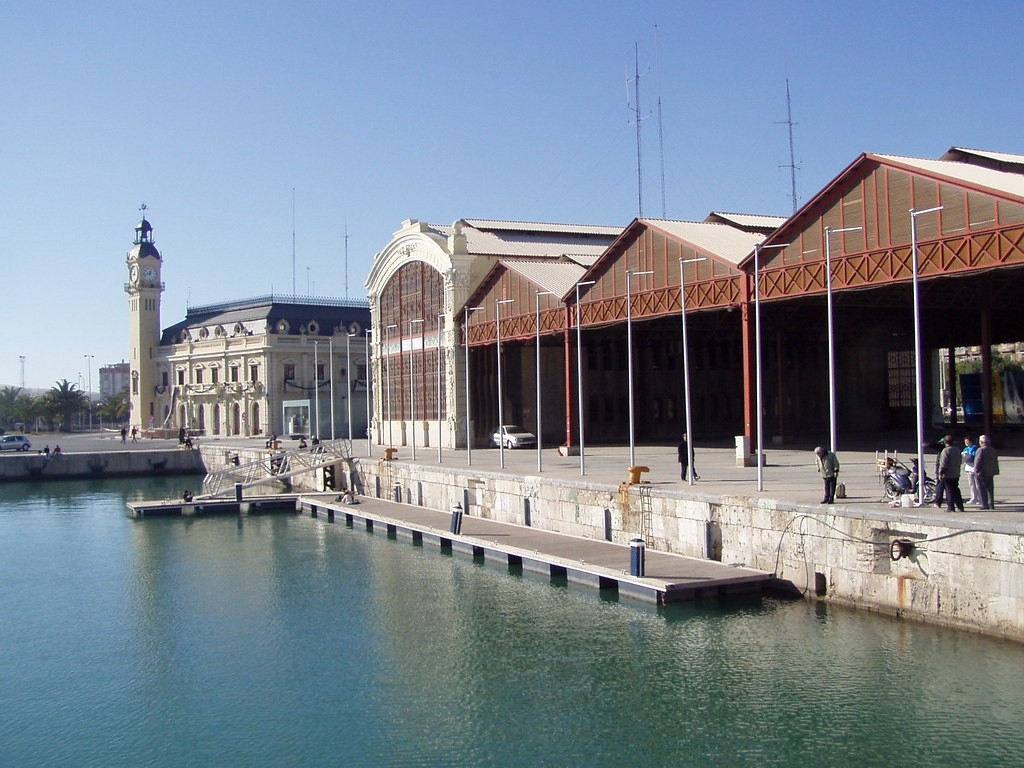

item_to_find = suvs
[0,434,33,451]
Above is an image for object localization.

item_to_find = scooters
[879,458,938,504]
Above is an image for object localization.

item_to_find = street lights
[83,354,95,430]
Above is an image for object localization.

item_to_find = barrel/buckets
[901,494,914,508]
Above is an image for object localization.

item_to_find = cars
[487,425,537,450]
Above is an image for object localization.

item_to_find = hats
[938,438,944,443]
[312,435,317,439]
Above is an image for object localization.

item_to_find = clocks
[143,265,157,281]
[129,266,139,284]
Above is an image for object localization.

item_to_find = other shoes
[933,503,942,509]
[977,506,990,510]
[682,478,687,481]
[966,499,976,505]
[695,476,700,481]
[945,507,956,512]
[820,499,828,504]
[828,500,834,504]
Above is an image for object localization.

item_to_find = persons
[298,438,307,448]
[265,431,276,448]
[341,488,354,504]
[131,426,138,443]
[183,490,193,502]
[934,435,964,512]
[120,425,126,444]
[179,427,185,445]
[310,435,319,453]
[293,415,308,435]
[961,436,979,505]
[679,434,700,482]
[974,435,1000,510]
[185,435,193,451]
[53,445,60,455]
[815,446,839,504]
[44,445,50,457]
